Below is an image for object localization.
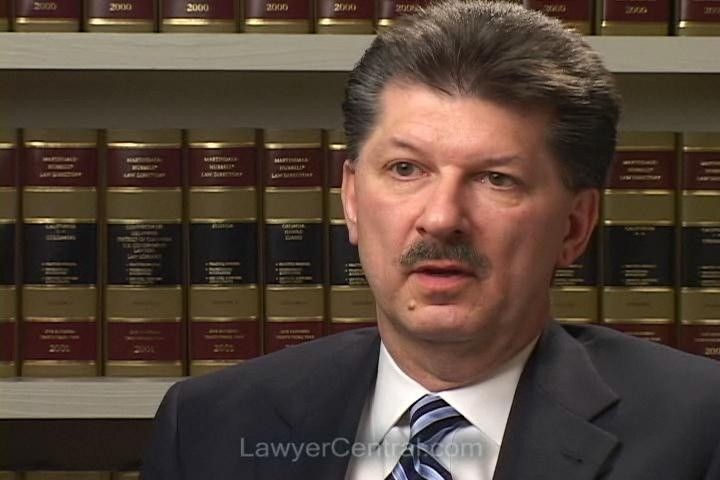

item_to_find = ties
[384,390,474,480]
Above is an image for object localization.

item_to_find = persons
[136,0,720,480]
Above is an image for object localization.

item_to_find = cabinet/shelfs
[0,32,719,418]
